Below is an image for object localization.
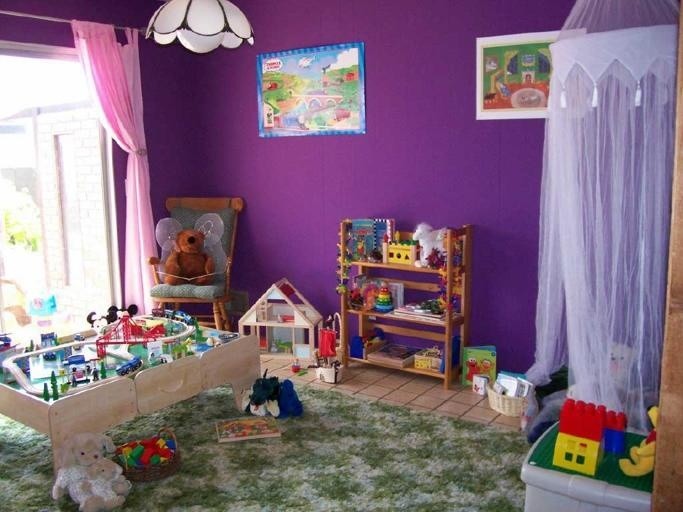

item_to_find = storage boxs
[520,410,651,511]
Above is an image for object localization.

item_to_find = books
[458,344,497,388]
[471,373,491,398]
[395,301,457,325]
[346,216,396,271]
[212,411,283,445]
[368,341,425,369]
[492,368,536,399]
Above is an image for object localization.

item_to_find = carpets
[0,376,531,512]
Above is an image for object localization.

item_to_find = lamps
[144,0,255,55]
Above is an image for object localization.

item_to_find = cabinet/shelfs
[339,217,472,389]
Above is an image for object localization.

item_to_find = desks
[0,315,259,496]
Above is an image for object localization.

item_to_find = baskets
[486,381,527,417]
[119,428,181,481]
[315,311,350,362]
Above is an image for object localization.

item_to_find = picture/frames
[256,42,365,138]
[475,28,587,121]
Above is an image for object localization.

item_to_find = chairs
[146,197,244,330]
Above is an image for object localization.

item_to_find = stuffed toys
[617,406,660,479]
[568,338,642,403]
[86,304,137,336]
[50,427,132,511]
[164,227,220,285]
